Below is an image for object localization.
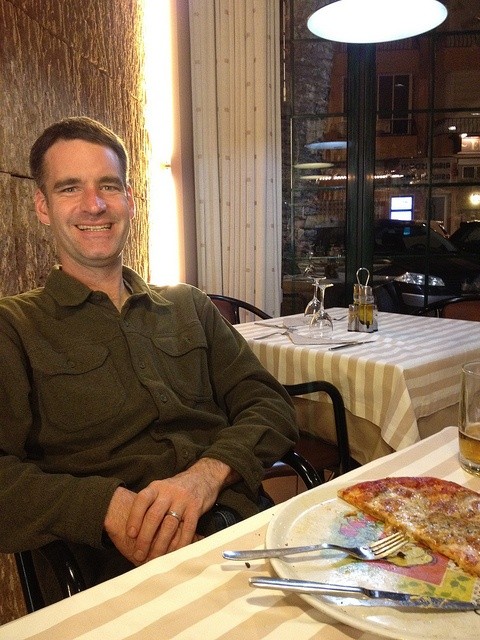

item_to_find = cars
[298,222,480,314]
[386,220,456,250]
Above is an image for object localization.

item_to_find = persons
[0,117,301,566]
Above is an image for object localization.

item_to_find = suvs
[448,219,480,253]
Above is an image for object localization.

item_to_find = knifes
[328,340,376,350]
[248,576,479,610]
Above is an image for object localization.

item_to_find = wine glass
[308,283,333,340]
[304,276,327,325]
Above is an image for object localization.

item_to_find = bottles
[356,286,378,333]
[347,284,363,332]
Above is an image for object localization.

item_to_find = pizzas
[338,477,480,582]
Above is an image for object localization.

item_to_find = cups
[457,360,480,478]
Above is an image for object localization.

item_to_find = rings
[166,510,182,521]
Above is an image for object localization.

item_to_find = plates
[265,480,479,640]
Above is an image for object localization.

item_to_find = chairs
[14,452,322,615]
[208,294,272,326]
[267,380,351,483]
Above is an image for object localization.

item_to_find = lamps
[305,1,449,45]
[294,154,334,169]
[304,124,347,150]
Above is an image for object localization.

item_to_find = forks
[221,530,410,561]
